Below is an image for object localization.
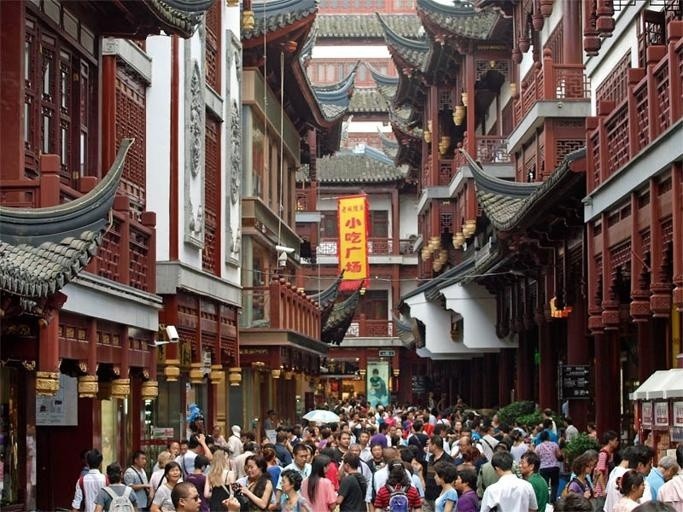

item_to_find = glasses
[177,494,200,501]
[585,464,597,469]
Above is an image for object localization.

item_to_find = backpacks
[100,485,135,512]
[561,477,594,498]
[383,483,413,512]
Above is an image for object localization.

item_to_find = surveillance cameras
[275,245,295,255]
[167,325,179,343]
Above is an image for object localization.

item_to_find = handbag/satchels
[234,493,250,512]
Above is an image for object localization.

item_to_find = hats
[195,454,208,465]
[231,425,241,438]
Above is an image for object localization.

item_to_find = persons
[370,367,388,404]
[68,387,683,512]
